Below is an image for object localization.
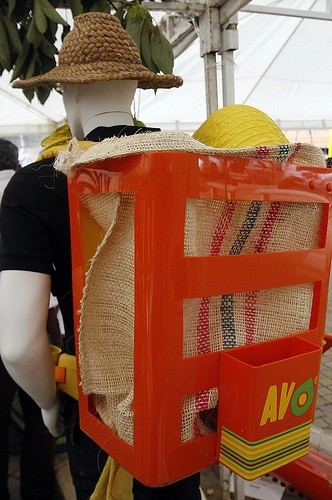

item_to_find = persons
[0,138,56,499]
[1,12,202,499]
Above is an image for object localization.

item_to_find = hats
[11,11,184,91]
